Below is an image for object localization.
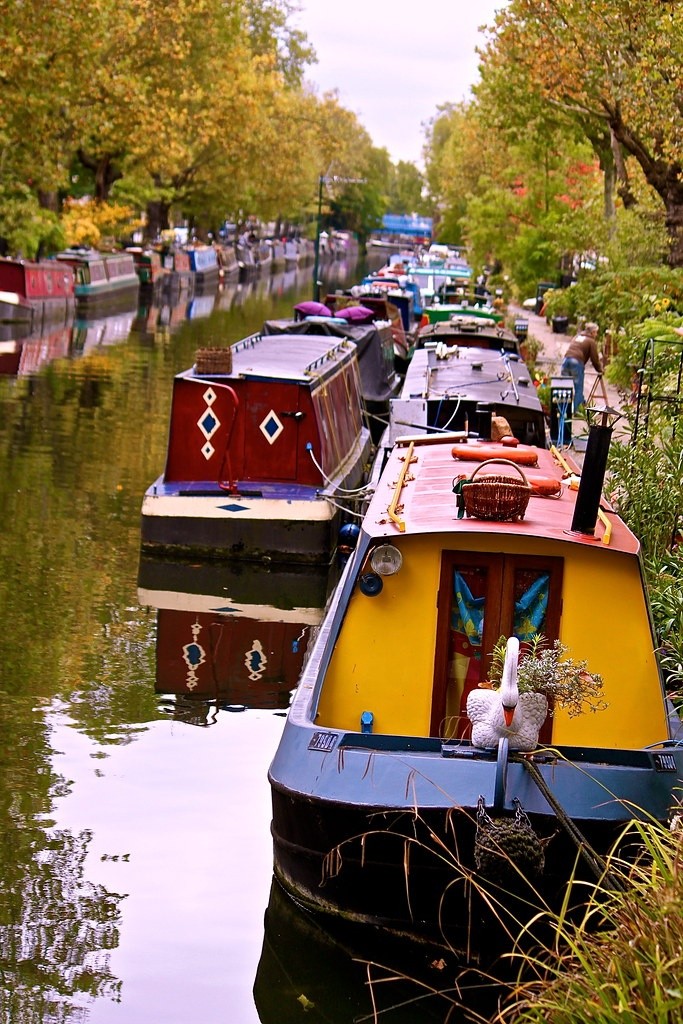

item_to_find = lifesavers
[459,474,563,495]
[451,445,539,466]
[372,281,399,289]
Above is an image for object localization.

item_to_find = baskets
[462,459,532,522]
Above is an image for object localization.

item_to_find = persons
[562,322,605,417]
[474,280,491,308]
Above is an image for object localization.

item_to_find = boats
[140,215,683,962]
[0,232,358,324]
[135,550,347,727]
[0,256,355,376]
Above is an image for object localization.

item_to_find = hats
[585,323,599,331]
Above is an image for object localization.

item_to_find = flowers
[479,633,610,719]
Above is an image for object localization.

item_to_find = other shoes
[575,413,582,418]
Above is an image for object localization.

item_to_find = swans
[465,636,549,752]
